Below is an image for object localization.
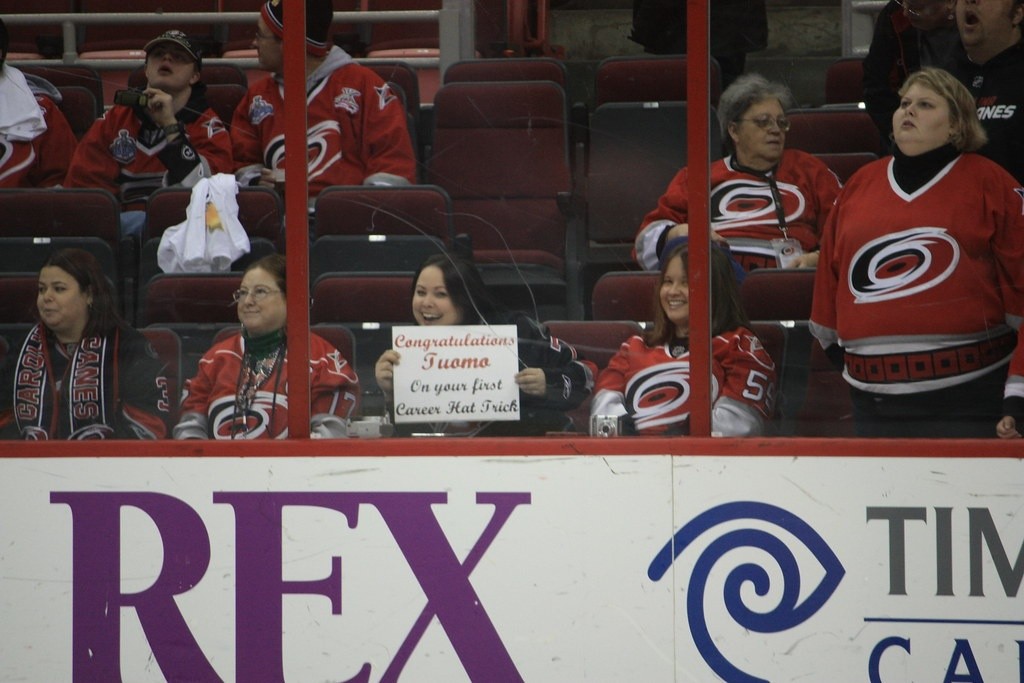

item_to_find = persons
[809,0,1024,439]
[0,18,78,189]
[589,236,778,438]
[628,0,768,87]
[632,72,844,271]
[375,251,598,437]
[0,248,170,440]
[172,254,361,440]
[229,0,416,241]
[65,28,236,240]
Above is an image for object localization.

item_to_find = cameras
[589,415,622,439]
[345,391,393,438]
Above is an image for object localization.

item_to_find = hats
[143,29,201,61]
[261,0,333,56]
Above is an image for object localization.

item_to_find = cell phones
[114,90,148,106]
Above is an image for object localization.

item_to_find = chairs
[0,0,880,438]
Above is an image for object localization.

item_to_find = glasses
[734,113,790,132]
[255,28,274,41]
[232,286,281,303]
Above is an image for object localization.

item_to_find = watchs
[163,120,186,136]
